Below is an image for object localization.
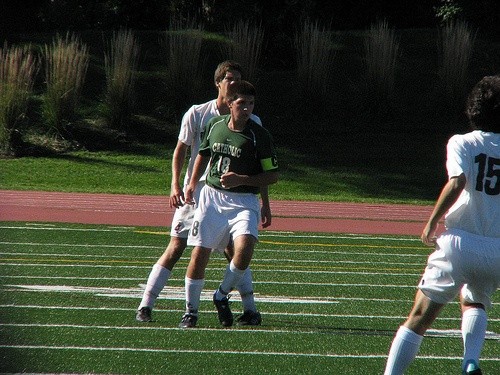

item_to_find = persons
[178,79,280,328]
[384,75,500,375]
[136,61,263,326]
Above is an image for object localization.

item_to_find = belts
[207,181,249,192]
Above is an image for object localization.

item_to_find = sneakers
[237,312,262,325]
[180,313,198,328]
[136,307,151,321]
[462,359,483,375]
[213,291,233,326]
